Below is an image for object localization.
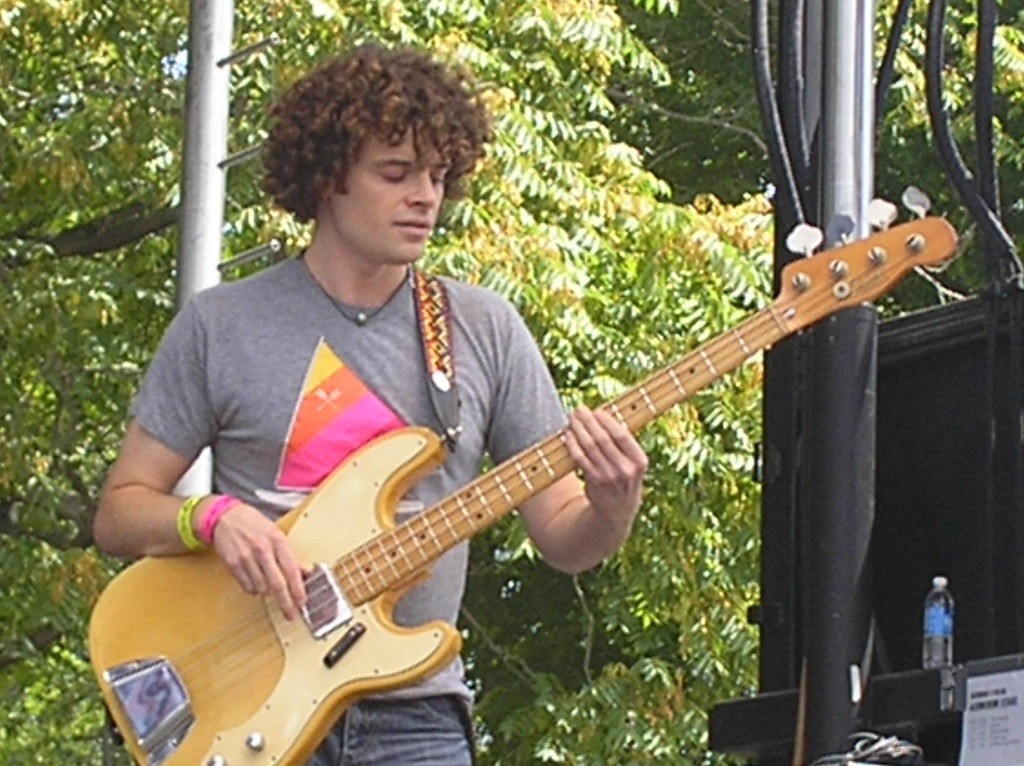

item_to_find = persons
[94,47,649,766]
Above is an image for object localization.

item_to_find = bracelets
[201,495,241,544]
[177,497,206,552]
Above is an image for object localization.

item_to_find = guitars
[88,184,956,766]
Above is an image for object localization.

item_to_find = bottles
[921,576,953,669]
